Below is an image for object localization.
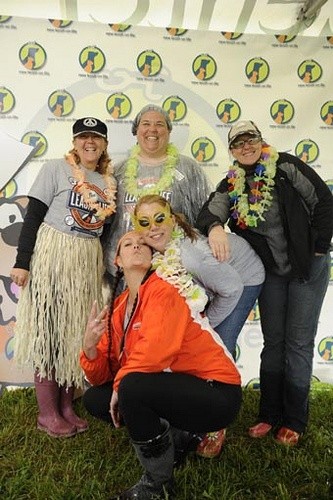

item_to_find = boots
[122,417,174,500]
[58,379,89,432]
[34,371,76,437]
[168,426,203,466]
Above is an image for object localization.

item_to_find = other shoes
[277,426,298,446]
[248,423,271,438]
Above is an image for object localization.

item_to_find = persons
[132,194,265,457]
[78,230,242,500]
[11,117,118,438]
[196,120,333,445]
[101,105,213,299]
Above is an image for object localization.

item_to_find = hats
[72,118,108,140]
[227,120,260,148]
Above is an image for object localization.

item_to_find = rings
[95,319,101,323]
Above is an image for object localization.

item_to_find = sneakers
[198,428,225,458]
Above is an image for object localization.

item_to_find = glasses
[231,138,260,149]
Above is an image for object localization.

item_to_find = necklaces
[226,142,279,230]
[123,141,179,200]
[149,224,209,312]
[64,152,117,220]
